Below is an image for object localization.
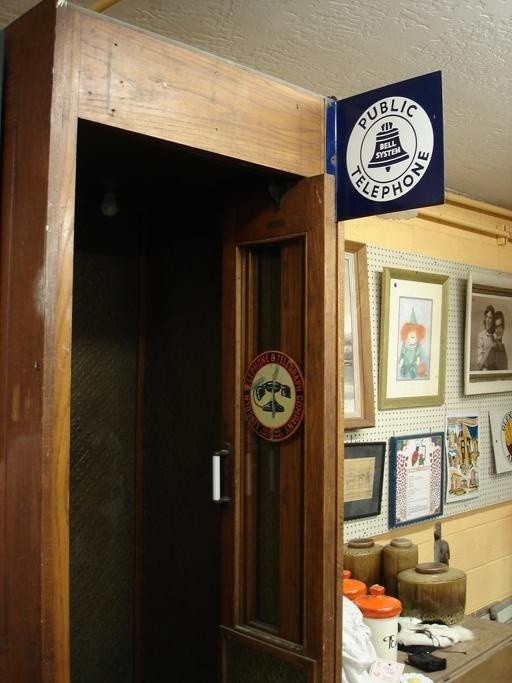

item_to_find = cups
[341,539,467,668]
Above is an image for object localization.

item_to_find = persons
[476,305,510,370]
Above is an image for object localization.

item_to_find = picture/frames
[464,270,512,396]
[343,442,386,520]
[379,267,450,410]
[344,239,375,432]
[390,432,443,528]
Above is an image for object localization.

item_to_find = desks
[395,613,512,683]
